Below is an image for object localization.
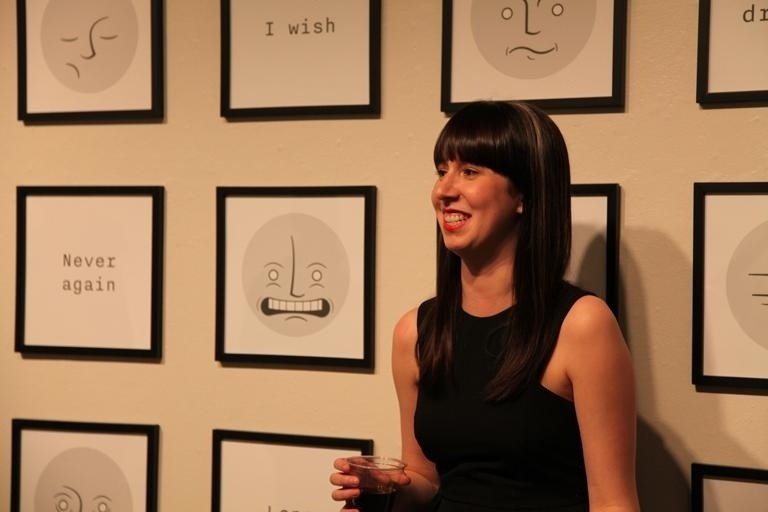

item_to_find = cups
[343,455,407,511]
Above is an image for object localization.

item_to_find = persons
[330,101,640,512]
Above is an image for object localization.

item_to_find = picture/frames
[219,0,382,120]
[439,0,629,116]
[691,180,768,390]
[14,185,164,360]
[436,182,621,323]
[690,462,768,511]
[10,417,159,512]
[211,428,374,512]
[695,1,768,106]
[15,0,165,126]
[215,185,377,374]
